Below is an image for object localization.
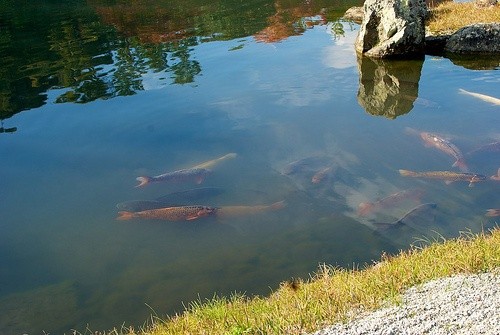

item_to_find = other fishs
[114,151,287,224]
[399,126,500,188]
[279,144,361,182]
[458,87,500,107]
[485,207,500,217]
[359,188,438,240]
